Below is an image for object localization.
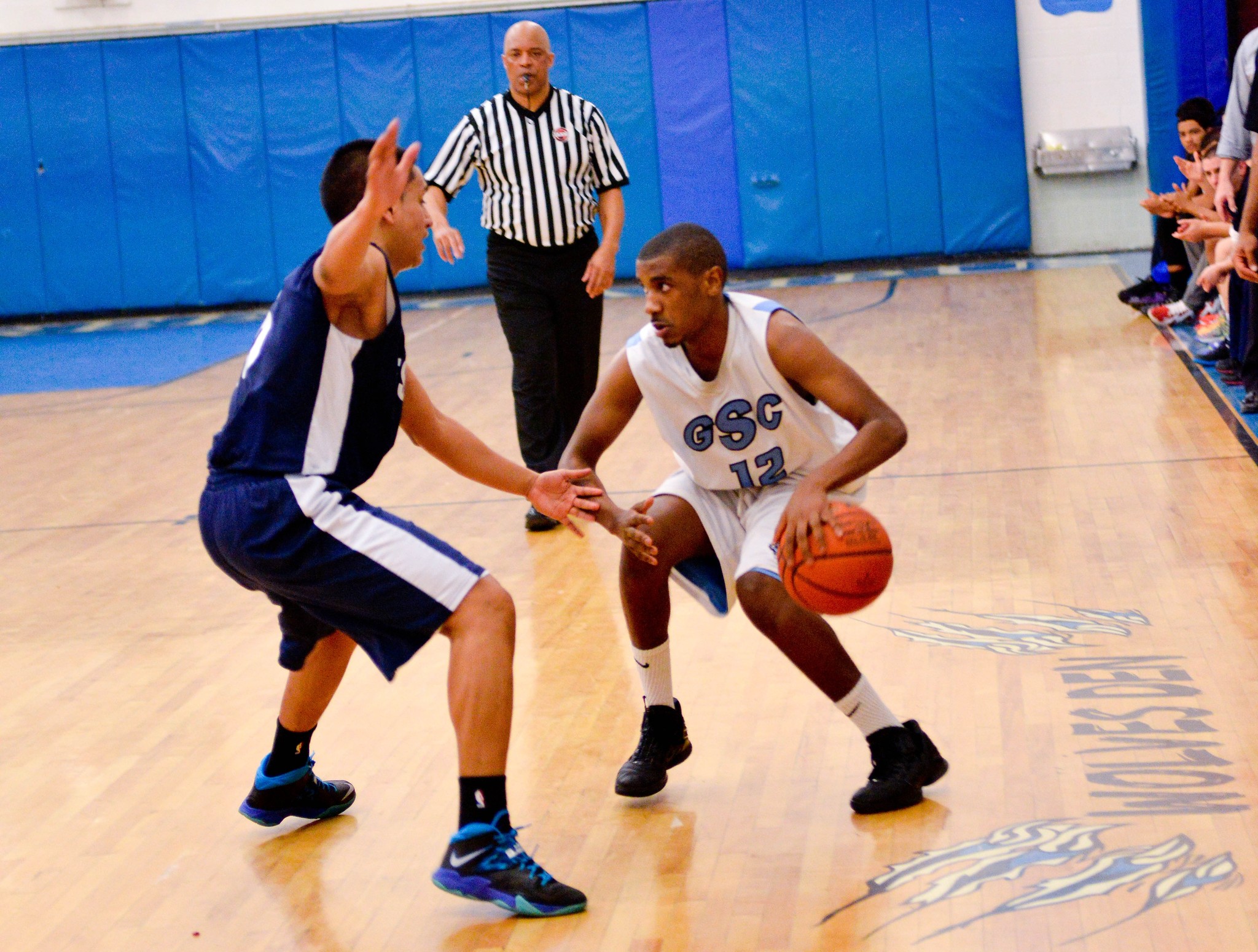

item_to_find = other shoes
[1215,358,1240,374]
[1240,391,1258,413]
[1196,315,1230,344]
[1220,374,1245,385]
[525,505,561,532]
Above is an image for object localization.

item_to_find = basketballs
[776,500,894,616]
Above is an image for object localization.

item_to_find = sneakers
[238,751,355,827]
[1197,298,1226,326]
[849,719,948,815]
[615,695,692,798]
[431,811,587,917]
[1147,299,1195,327]
[1118,277,1181,305]
[1193,336,1231,366]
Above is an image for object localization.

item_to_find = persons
[558,223,948,814]
[1117,27,1258,414]
[423,20,630,533]
[198,119,603,917]
